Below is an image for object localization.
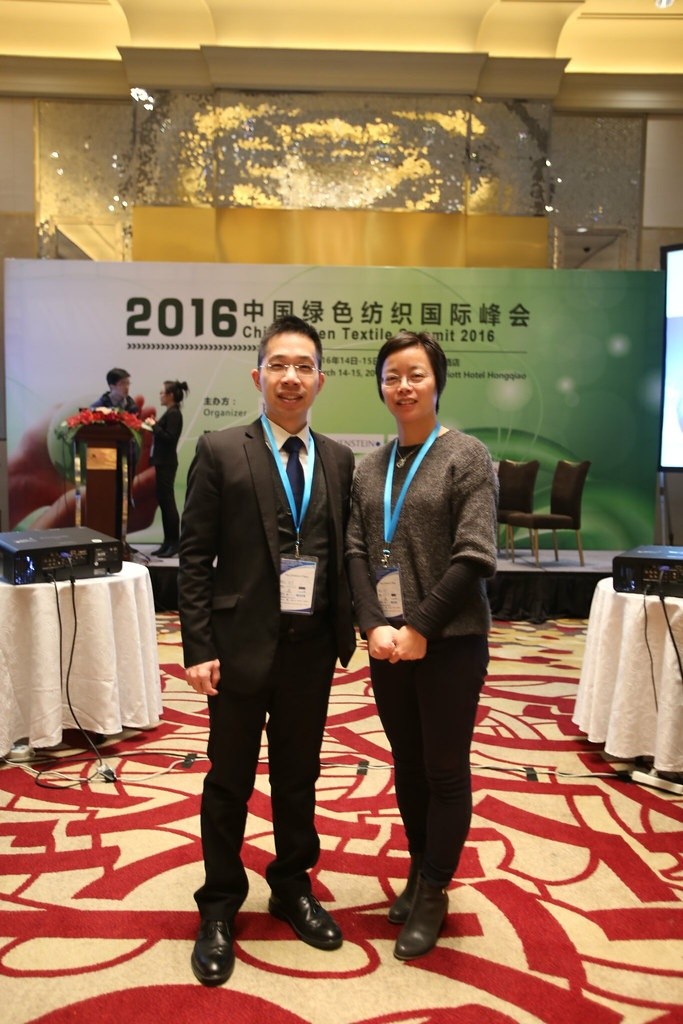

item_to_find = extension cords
[4,744,31,760]
[632,770,683,795]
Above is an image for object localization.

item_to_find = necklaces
[395,440,426,468]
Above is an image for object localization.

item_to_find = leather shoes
[191,918,235,985]
[269,892,343,949]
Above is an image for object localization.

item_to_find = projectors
[612,544,683,598]
[0,526,123,587]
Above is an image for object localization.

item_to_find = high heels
[151,539,180,559]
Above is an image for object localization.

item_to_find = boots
[389,868,422,926]
[395,877,449,961]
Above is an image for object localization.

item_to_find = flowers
[57,408,144,449]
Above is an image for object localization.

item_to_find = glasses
[259,361,323,377]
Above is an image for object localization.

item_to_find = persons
[346,331,499,960]
[89,368,142,420]
[178,316,355,987]
[146,381,189,559]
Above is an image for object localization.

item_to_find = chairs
[496,460,541,556]
[507,458,592,566]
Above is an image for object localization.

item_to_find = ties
[282,436,305,526]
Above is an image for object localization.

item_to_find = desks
[571,577,683,777]
[0,561,163,758]
